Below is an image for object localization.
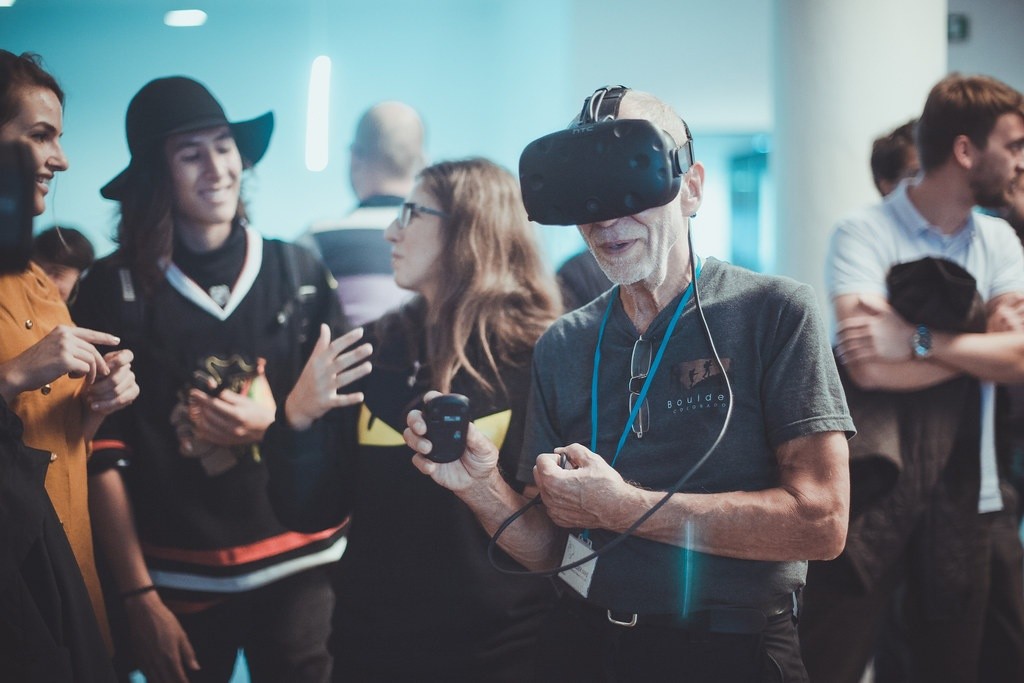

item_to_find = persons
[293,100,423,333]
[403,87,857,683]
[65,76,356,683]
[0,51,141,683]
[258,158,613,683]
[798,74,1024,683]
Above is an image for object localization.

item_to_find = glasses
[629,334,654,439]
[397,202,449,229]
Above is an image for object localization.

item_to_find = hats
[99,76,275,202]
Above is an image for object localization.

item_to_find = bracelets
[121,585,157,599]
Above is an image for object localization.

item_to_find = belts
[605,593,798,629]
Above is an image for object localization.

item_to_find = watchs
[912,325,933,359]
